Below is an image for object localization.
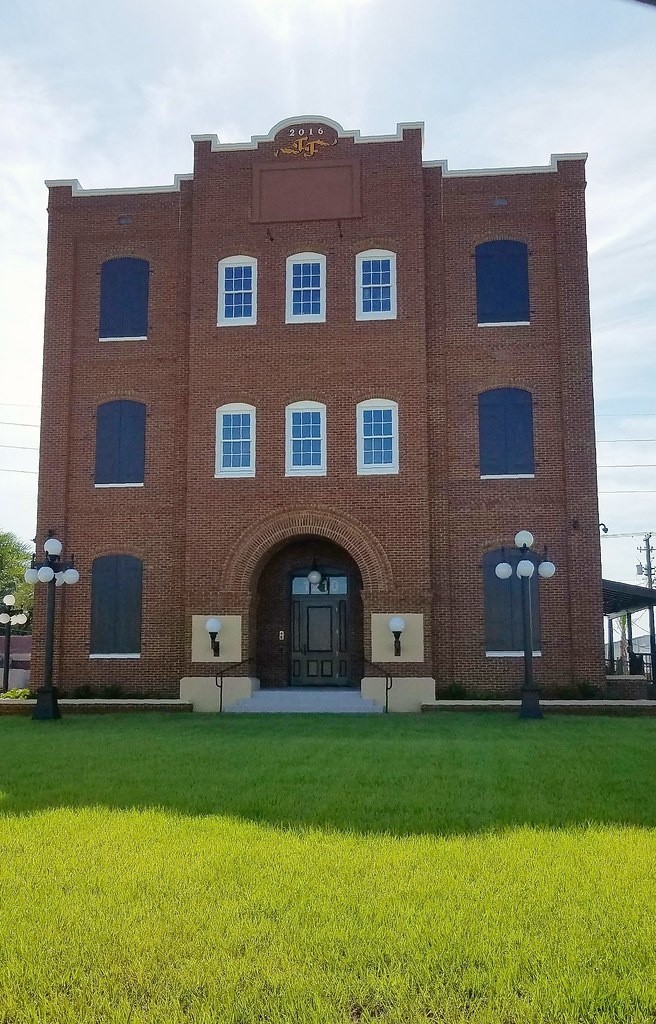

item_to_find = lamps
[387,617,404,656]
[308,557,322,584]
[206,619,221,657]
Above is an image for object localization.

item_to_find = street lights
[0,595,27,694]
[636,539,656,684]
[495,530,555,720]
[24,538,79,721]
